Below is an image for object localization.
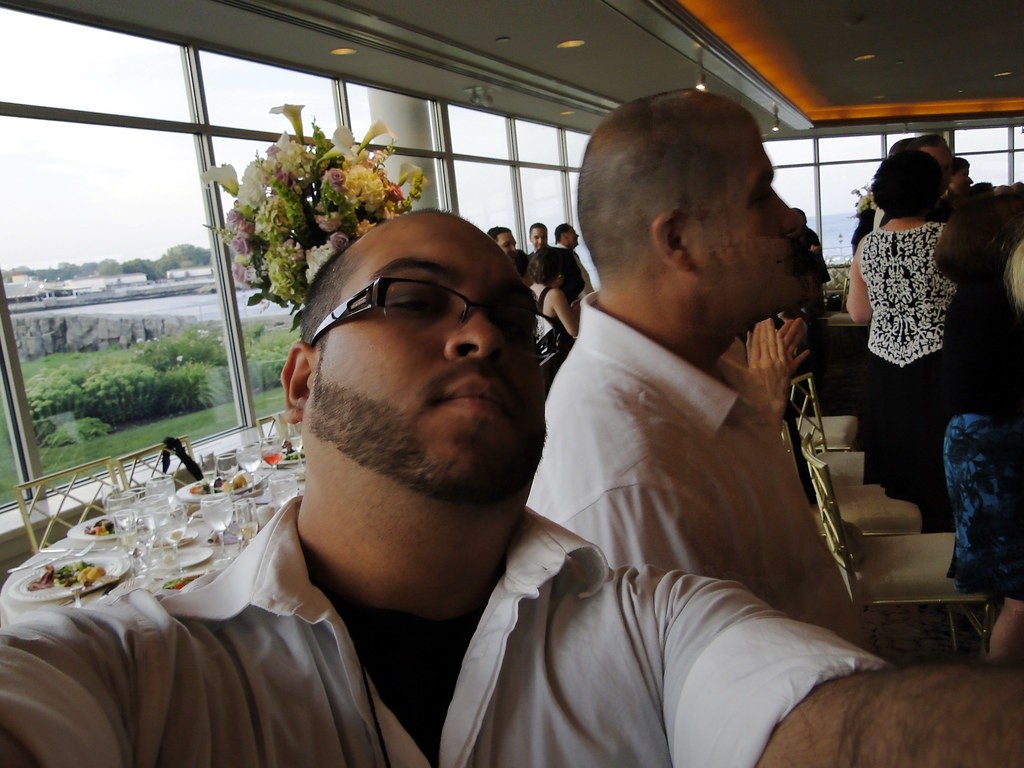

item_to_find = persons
[486,223,595,402]
[524,88,873,657]
[0,209,1022,767]
[719,135,1023,660]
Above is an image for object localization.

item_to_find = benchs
[820,265,874,354]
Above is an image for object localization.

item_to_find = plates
[156,546,214,571]
[153,531,198,550]
[276,457,305,465]
[162,572,204,595]
[67,515,135,540]
[109,574,165,603]
[8,556,130,601]
[173,472,262,501]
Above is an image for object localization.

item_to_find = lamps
[695,64,706,91]
[770,113,780,132]
[461,86,499,115]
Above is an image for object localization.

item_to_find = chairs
[256,411,302,441]
[12,456,121,555]
[115,434,204,499]
[780,371,994,657]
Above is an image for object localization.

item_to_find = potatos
[77,566,105,582]
[232,474,247,489]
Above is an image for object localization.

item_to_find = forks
[7,542,96,572]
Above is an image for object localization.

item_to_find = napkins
[202,522,244,546]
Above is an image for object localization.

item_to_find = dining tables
[0,459,307,630]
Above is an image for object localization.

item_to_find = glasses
[310,275,560,367]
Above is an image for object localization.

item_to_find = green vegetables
[162,575,192,590]
[93,518,115,534]
[283,453,306,459]
[57,561,93,584]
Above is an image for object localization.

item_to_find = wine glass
[104,422,303,580]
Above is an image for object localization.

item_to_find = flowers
[200,104,428,333]
[847,183,877,218]
